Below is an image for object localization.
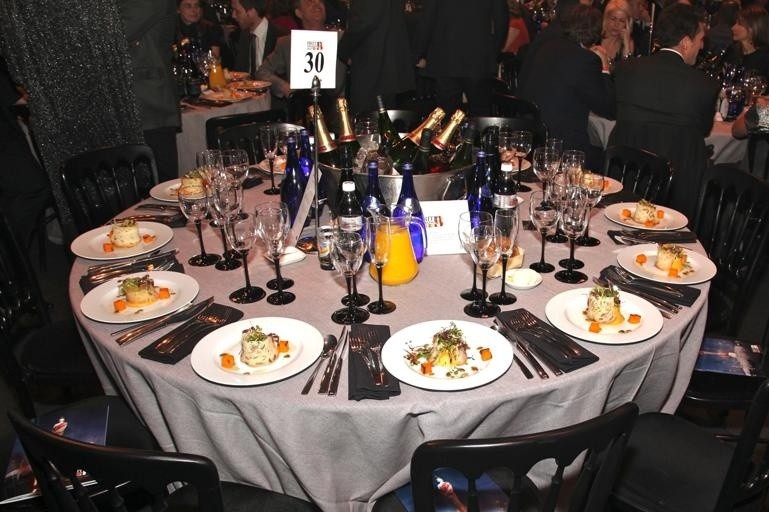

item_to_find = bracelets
[602,66,610,71]
[623,51,630,56]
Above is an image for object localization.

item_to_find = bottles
[429,109,465,156]
[308,103,342,168]
[694,50,711,71]
[280,136,312,228]
[362,159,390,264]
[297,129,325,217]
[429,143,457,175]
[335,154,364,210]
[720,64,746,121]
[379,107,445,175]
[493,162,518,237]
[449,121,476,168]
[337,180,366,272]
[169,44,187,96]
[393,162,424,264]
[184,49,201,97]
[373,92,403,152]
[412,127,433,174]
[467,150,492,238]
[717,63,732,113]
[333,98,361,169]
[360,130,393,175]
[705,49,727,77]
[484,133,501,178]
[487,125,499,137]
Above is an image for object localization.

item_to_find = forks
[347,330,380,386]
[355,327,379,384]
[166,308,232,353]
[500,322,548,379]
[590,277,683,319]
[133,203,180,212]
[620,229,684,240]
[613,266,683,298]
[154,307,212,350]
[87,249,175,286]
[602,269,680,297]
[509,316,572,360]
[366,329,383,384]
[520,311,582,356]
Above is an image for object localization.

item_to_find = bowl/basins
[315,132,476,217]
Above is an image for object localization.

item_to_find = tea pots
[368,204,429,288]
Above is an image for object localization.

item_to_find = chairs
[0,211,105,422]
[472,94,549,165]
[370,401,640,512]
[599,144,674,210]
[610,379,769,512]
[3,405,319,512]
[57,142,160,235]
[694,161,769,338]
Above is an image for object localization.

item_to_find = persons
[612,3,718,176]
[162,0,227,76]
[221,1,292,79]
[719,15,769,87]
[337,1,426,111]
[260,2,348,96]
[598,0,638,58]
[501,0,530,55]
[420,1,508,114]
[518,0,608,184]
[711,97,768,277]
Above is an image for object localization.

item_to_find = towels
[138,303,243,364]
[80,253,184,295]
[596,190,640,208]
[241,176,262,188]
[158,215,187,227]
[348,324,400,400]
[607,230,697,243]
[497,308,599,372]
[513,168,540,182]
[600,262,701,306]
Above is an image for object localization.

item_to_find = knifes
[328,329,349,394]
[110,296,213,346]
[318,325,345,393]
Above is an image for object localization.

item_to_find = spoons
[301,334,337,395]
[489,325,533,379]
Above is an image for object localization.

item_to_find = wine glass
[458,209,493,301]
[222,212,265,304]
[207,176,249,271]
[544,139,562,160]
[553,195,588,283]
[260,207,294,290]
[332,213,370,307]
[366,215,396,315]
[561,150,585,176]
[489,208,519,306]
[528,191,554,273]
[259,125,281,196]
[575,172,600,247]
[254,198,296,306]
[329,232,369,325]
[464,225,501,318]
[510,130,532,192]
[745,68,765,104]
[532,147,559,211]
[177,185,221,266]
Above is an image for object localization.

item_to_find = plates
[553,172,623,194]
[70,221,172,260]
[201,90,255,103]
[188,315,323,387]
[604,202,689,229]
[381,317,514,392]
[79,270,199,324]
[263,245,305,265]
[616,243,718,285]
[503,268,542,290]
[259,155,285,174]
[149,177,205,202]
[226,81,273,93]
[508,157,532,173]
[546,286,664,344]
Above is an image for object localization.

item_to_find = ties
[251,33,256,73]
